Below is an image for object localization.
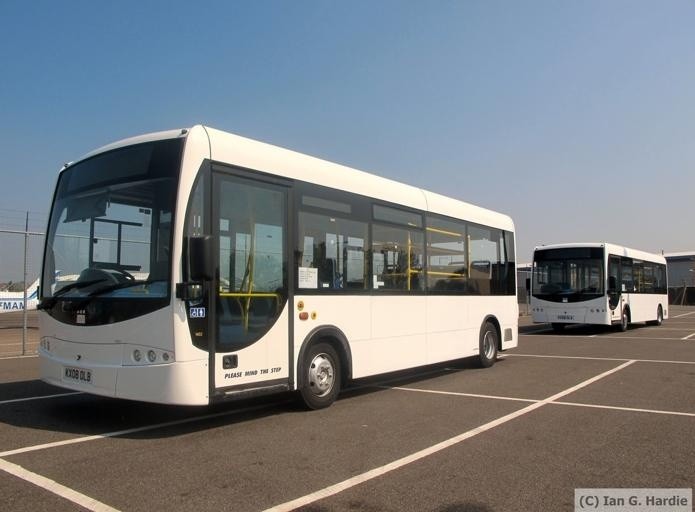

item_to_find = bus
[527,240,671,333]
[37,122,519,411]
